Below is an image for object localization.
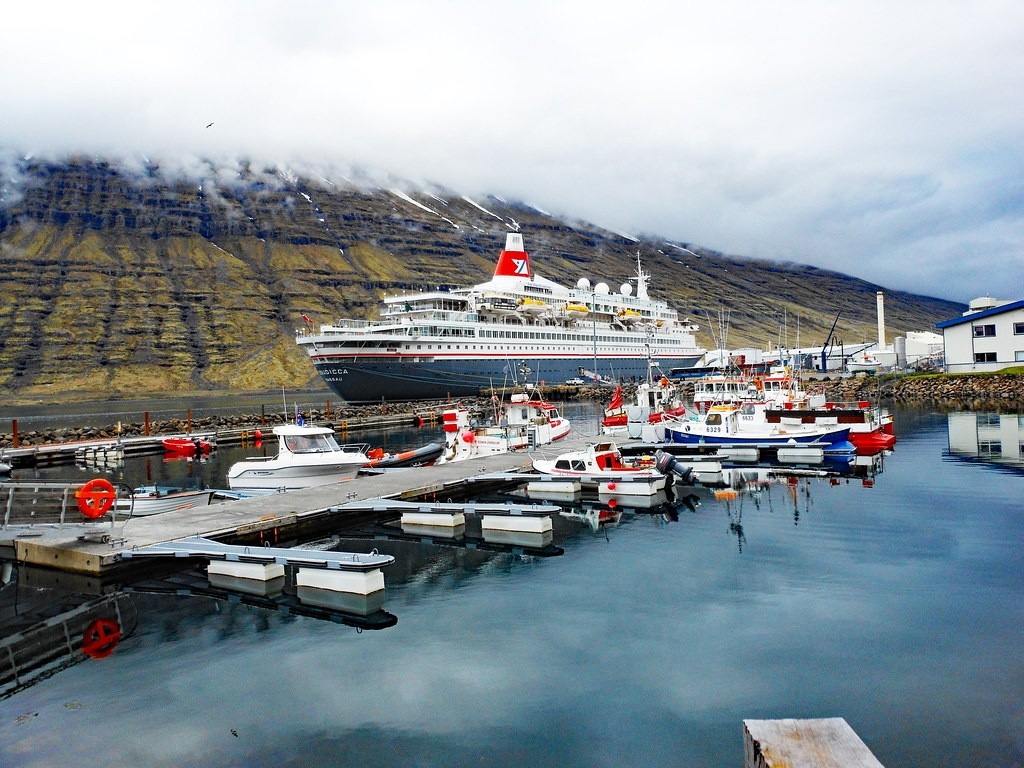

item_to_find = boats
[517,300,898,489]
[425,354,573,466]
[617,309,643,325]
[565,304,590,319]
[86,481,217,519]
[226,386,371,493]
[160,435,213,461]
[357,442,445,468]
[514,297,548,315]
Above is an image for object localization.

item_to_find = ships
[292,220,711,407]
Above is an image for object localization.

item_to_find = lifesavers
[83,621,120,659]
[77,479,115,517]
